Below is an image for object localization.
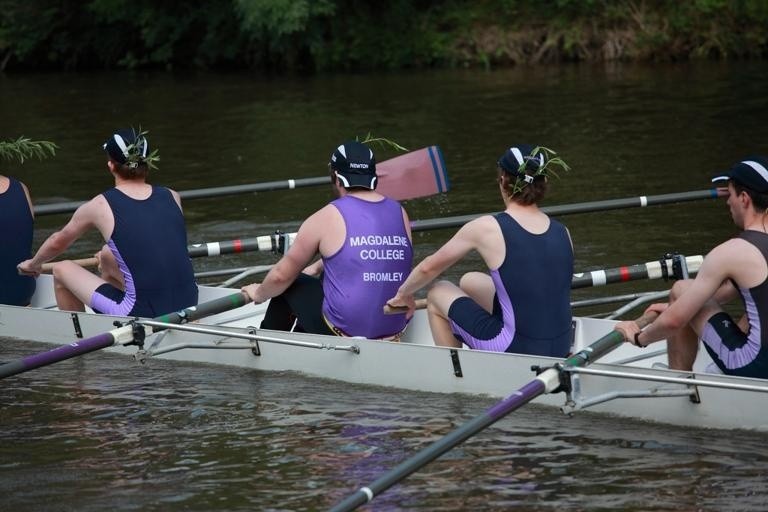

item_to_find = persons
[0,174,35,306]
[240,142,413,341]
[617,161,768,379]
[15,128,199,316]
[388,145,576,358]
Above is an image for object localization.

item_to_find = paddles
[32,144,449,216]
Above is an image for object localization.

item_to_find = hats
[496,143,547,183]
[329,142,379,191]
[102,127,150,169]
[711,159,768,195]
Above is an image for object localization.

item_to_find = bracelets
[634,331,648,349]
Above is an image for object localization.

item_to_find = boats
[1,267,768,433]
